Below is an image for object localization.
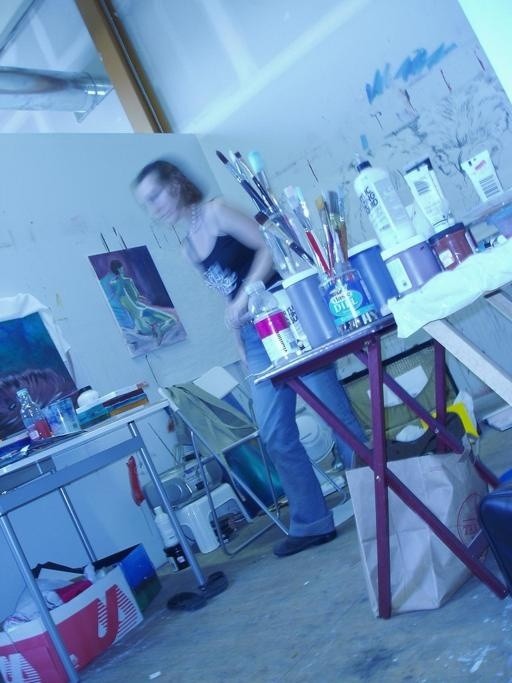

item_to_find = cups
[41,397,82,435]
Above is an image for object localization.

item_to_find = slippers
[191,571,228,599]
[166,592,206,611]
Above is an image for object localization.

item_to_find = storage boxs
[91,542,163,612]
[1,564,146,682]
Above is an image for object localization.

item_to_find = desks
[1,400,205,681]
[252,279,511,619]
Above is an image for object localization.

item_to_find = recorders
[143,455,222,515]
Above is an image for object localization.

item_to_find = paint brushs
[216,150,348,279]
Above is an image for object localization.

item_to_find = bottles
[14,386,52,442]
[152,505,178,547]
[355,160,414,250]
[244,201,399,369]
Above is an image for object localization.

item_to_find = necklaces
[187,200,206,236]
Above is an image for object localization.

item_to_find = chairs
[156,364,348,557]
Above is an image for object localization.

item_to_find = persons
[109,258,176,348]
[130,158,369,558]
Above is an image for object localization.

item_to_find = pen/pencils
[247,151,303,250]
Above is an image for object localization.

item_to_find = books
[75,381,150,429]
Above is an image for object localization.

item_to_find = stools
[167,481,253,553]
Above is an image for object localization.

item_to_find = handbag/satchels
[345,432,486,618]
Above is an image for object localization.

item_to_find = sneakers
[273,531,336,557]
